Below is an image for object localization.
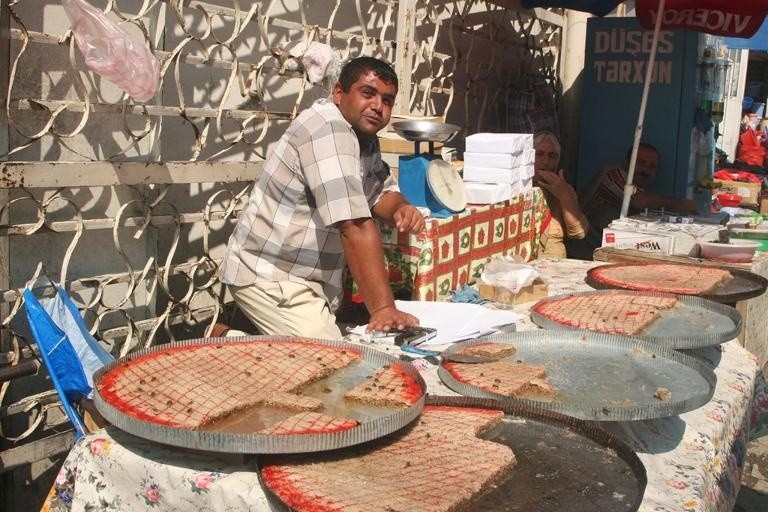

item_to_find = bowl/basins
[695,239,762,263]
[717,194,742,207]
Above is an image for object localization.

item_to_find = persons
[578,143,698,248]
[532,131,590,260]
[204,56,426,343]
[740,114,768,141]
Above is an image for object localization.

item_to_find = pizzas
[534,293,678,336]
[442,343,555,397]
[98,342,420,434]
[592,263,735,294]
[261,404,518,512]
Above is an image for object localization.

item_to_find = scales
[391,120,468,218]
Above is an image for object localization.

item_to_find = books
[345,324,437,348]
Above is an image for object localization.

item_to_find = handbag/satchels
[504,73,561,143]
